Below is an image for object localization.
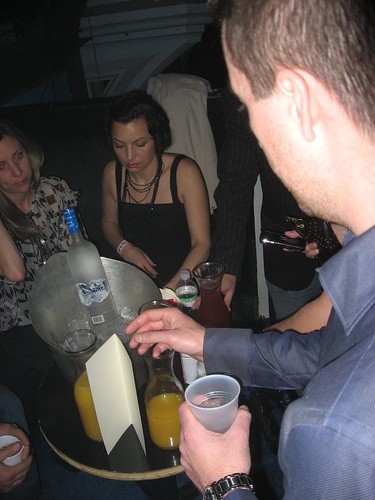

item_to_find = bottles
[63,329,103,442]
[192,261,231,328]
[62,207,118,326]
[174,269,198,318]
[138,299,184,451]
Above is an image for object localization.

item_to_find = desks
[36,359,200,500]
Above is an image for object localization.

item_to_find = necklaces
[126,176,155,192]
[128,172,154,185]
[126,184,151,203]
[134,187,149,190]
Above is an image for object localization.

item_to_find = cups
[0,435,24,466]
[184,373,241,435]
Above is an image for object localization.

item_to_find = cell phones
[259,231,306,251]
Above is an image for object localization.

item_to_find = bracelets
[115,239,130,255]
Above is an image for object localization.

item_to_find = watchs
[202,473,253,500]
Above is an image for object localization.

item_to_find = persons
[0,89,350,500]
[123,0,375,500]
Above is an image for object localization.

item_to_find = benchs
[0,89,227,221]
